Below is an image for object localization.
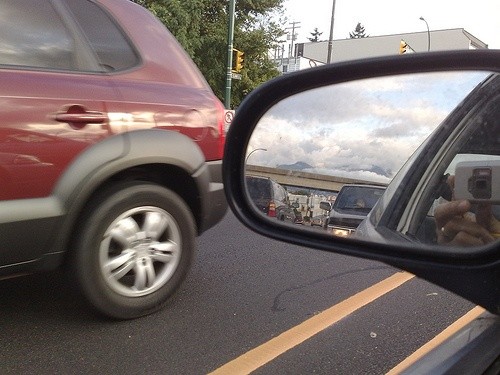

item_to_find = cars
[311,218,323,227]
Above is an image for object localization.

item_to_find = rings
[441,225,450,239]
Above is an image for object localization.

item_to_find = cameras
[453,161,500,205]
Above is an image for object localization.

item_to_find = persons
[427,174,500,247]
[348,195,366,209]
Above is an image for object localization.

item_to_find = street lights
[245,148,267,174]
[420,16,431,52]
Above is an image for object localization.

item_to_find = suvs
[0,0,229,320]
[321,184,387,237]
[246,174,300,224]
